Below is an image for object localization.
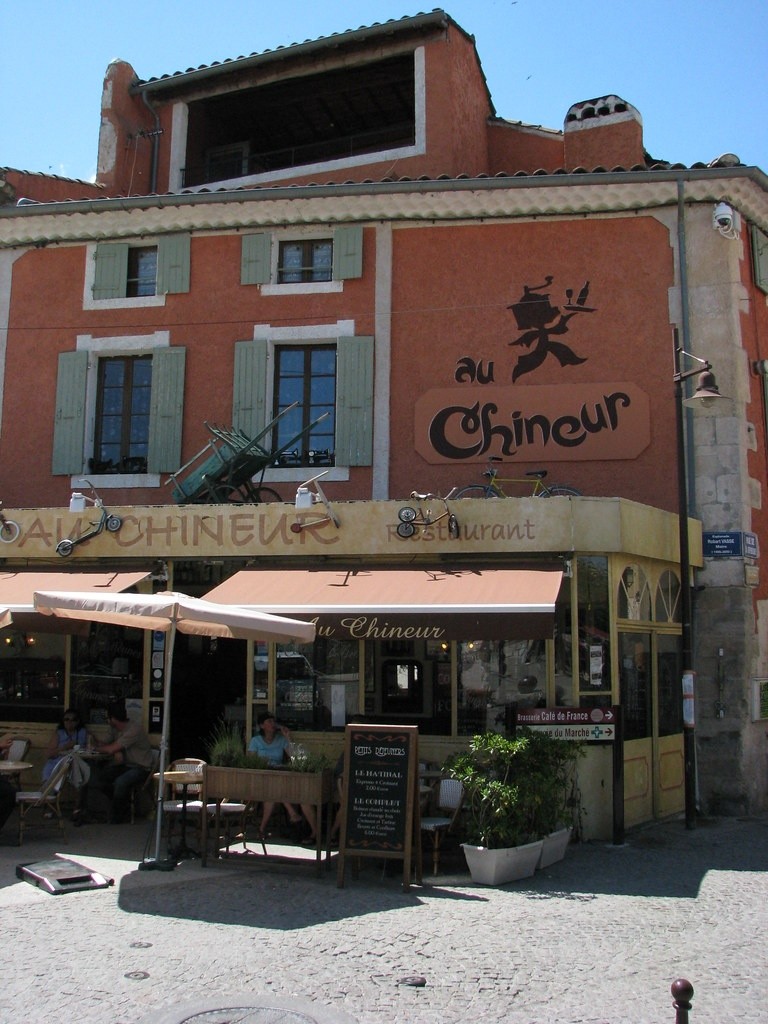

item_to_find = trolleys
[162,400,331,507]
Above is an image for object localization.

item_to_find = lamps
[670,347,735,410]
[296,488,322,509]
[67,493,103,513]
[6,632,38,653]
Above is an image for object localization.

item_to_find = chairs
[129,745,163,826]
[15,756,74,847]
[0,736,32,793]
[420,778,468,877]
[143,758,267,862]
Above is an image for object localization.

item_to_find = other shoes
[301,836,316,844]
[256,830,265,841]
[330,836,339,847]
[291,814,301,824]
[44,812,52,819]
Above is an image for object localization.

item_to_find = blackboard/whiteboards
[341,724,419,859]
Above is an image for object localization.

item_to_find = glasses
[64,717,80,721]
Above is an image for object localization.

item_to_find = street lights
[667,322,734,826]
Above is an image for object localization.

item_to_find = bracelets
[93,744,98,750]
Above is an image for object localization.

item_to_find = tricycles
[395,487,460,538]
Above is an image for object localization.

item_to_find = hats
[257,711,274,724]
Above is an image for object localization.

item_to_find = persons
[72,704,153,827]
[328,713,368,849]
[245,710,303,841]
[40,709,93,819]
[0,732,13,748]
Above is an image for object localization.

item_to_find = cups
[87,735,92,753]
[73,744,80,751]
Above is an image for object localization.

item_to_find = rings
[7,738,9,743]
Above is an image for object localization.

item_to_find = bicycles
[1,497,21,544]
[453,455,583,499]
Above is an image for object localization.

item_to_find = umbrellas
[31,589,318,867]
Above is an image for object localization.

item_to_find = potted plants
[444,731,588,887]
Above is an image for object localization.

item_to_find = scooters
[290,470,341,533]
[55,477,123,558]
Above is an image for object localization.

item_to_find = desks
[0,760,35,772]
[418,771,450,778]
[153,770,204,861]
[59,748,114,826]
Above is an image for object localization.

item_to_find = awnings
[197,558,567,642]
[0,568,154,613]
